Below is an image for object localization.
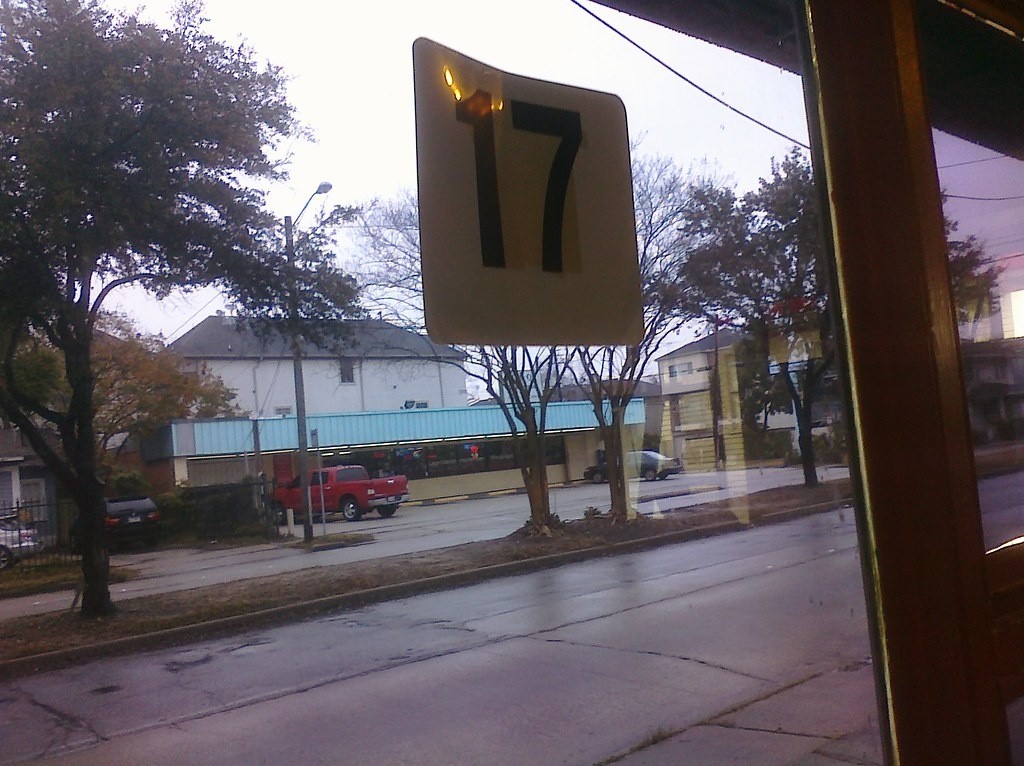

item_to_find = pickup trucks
[272,464,413,527]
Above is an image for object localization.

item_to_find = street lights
[284,182,332,542]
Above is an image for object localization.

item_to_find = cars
[70,494,161,555]
[0,515,39,571]
[584,451,683,485]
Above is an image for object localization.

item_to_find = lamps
[415,400,428,408]
[273,405,293,417]
[405,399,416,409]
